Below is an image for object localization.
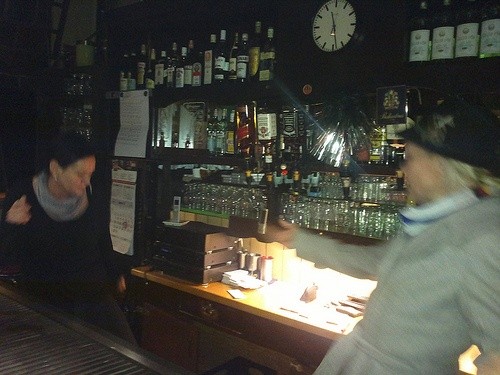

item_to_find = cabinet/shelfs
[96,0,500,267]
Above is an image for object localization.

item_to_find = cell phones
[172,196,181,223]
[257,208,269,235]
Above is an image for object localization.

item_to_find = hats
[395,92,500,179]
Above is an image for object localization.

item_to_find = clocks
[308,0,366,58]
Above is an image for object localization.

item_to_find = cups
[181,171,405,241]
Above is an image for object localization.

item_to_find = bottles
[237,105,254,159]
[480,0,500,60]
[454,0,479,60]
[217,109,228,155]
[121,20,276,91]
[340,160,352,199]
[226,109,237,155]
[409,0,430,64]
[265,174,279,223]
[432,0,454,63]
[289,169,305,195]
[206,108,218,154]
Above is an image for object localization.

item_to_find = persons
[0,132,139,351]
[253,93,500,375]
